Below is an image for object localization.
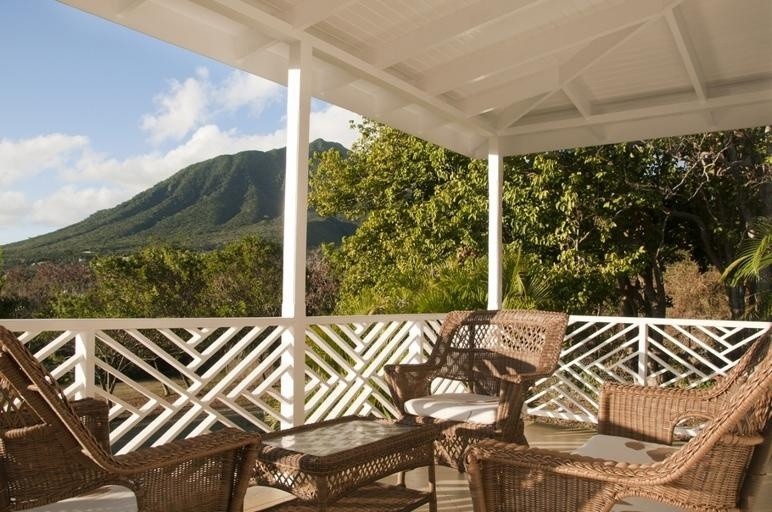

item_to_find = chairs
[381,308,570,476]
[0,322,265,511]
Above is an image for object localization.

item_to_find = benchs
[452,323,771,512]
